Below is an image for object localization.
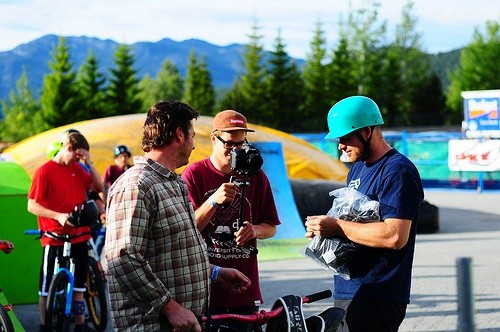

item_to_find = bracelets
[211,265,219,283]
[87,161,93,168]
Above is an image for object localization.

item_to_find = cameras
[229,144,263,172]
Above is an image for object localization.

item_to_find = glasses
[216,135,245,149]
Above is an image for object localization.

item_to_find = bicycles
[0,244,25,332]
[24,224,109,332]
[174,289,334,332]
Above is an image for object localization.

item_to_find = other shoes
[74,323,95,332]
[39,324,46,332]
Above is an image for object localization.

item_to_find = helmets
[265,295,349,332]
[70,199,103,231]
[323,96,384,141]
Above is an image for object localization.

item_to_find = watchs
[209,196,219,207]
[211,267,224,284]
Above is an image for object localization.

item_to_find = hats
[113,145,131,159]
[212,109,255,133]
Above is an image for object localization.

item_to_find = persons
[104,100,252,332]
[105,145,133,191]
[181,110,281,332]
[54,129,106,322]
[304,96,424,332]
[27,132,105,332]
